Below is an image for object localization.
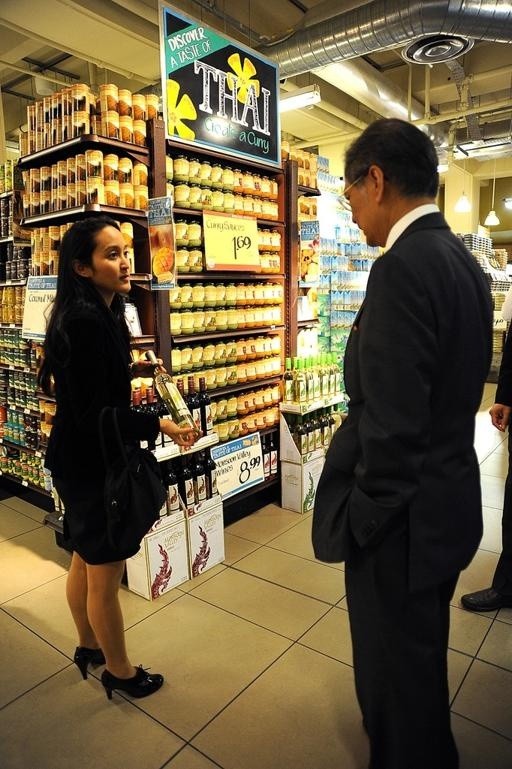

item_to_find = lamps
[484,154,502,226]
[198,0,322,115]
[454,151,473,214]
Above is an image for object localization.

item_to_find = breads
[152,247,175,274]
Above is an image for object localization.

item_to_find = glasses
[337,173,364,212]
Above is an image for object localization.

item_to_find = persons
[35,217,205,702]
[459,279,512,610]
[308,117,493,768]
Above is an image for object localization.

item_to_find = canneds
[166,151,284,443]
[0,159,79,490]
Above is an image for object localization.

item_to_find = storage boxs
[183,501,227,580]
[280,455,327,515]
[125,517,190,602]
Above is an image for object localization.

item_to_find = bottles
[0,283,61,499]
[132,376,214,451]
[156,432,279,519]
[167,278,283,440]
[281,351,339,404]
[1,83,285,280]
[144,348,200,439]
[284,403,344,457]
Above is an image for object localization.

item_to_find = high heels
[73,646,107,680]
[101,664,165,701]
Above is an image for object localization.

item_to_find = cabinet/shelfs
[18,113,175,550]
[289,159,323,369]
[159,134,294,526]
[0,184,51,510]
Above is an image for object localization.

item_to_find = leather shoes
[461,587,512,613]
